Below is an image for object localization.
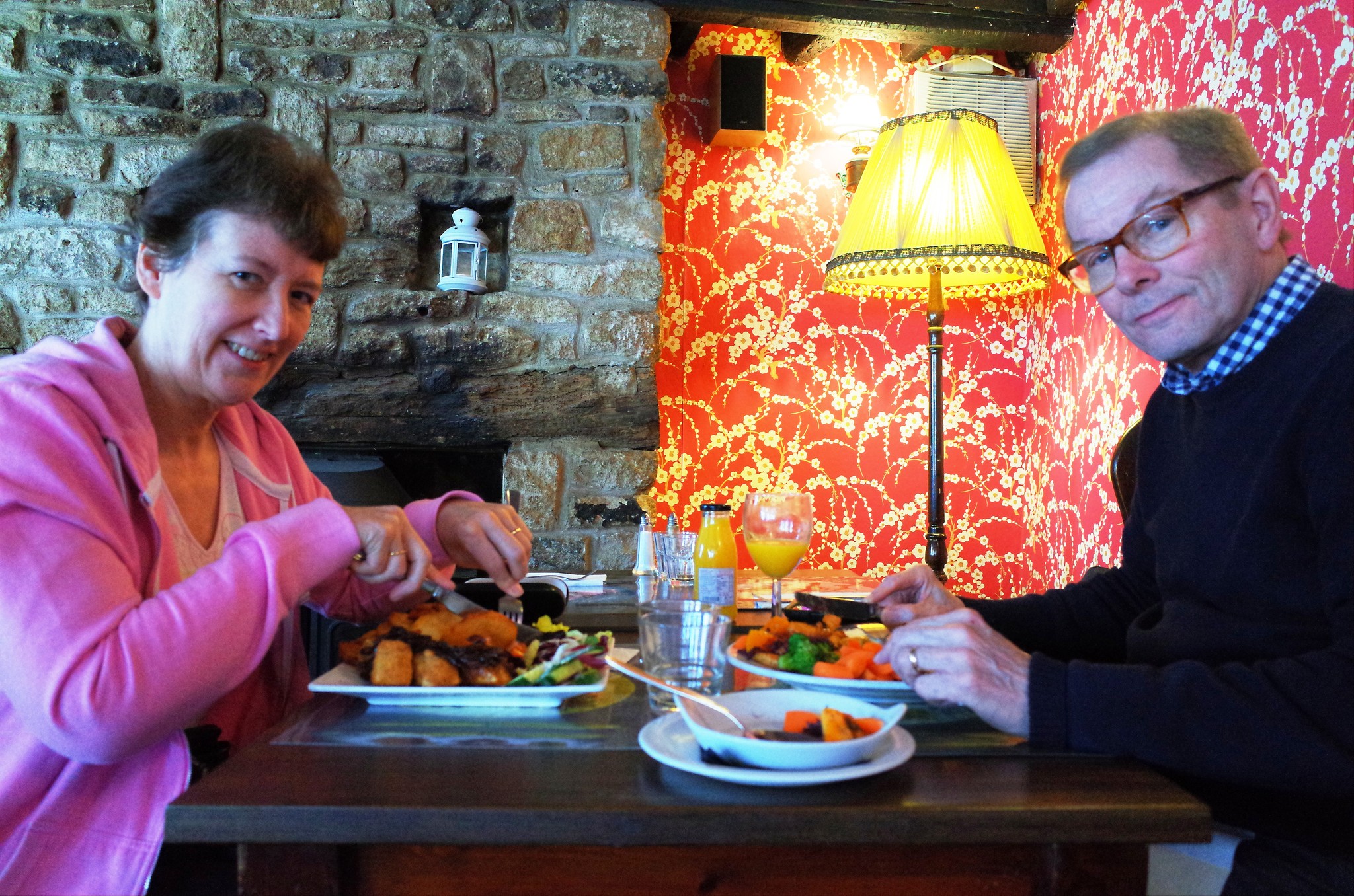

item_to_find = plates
[637,712,916,787]
[307,636,615,708]
[727,643,928,703]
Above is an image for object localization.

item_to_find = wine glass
[743,492,814,618]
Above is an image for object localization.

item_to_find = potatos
[783,706,881,742]
[337,602,528,686]
[734,610,903,681]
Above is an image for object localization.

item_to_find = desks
[409,534,880,630]
[147,629,1222,895]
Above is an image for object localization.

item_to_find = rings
[509,527,521,534]
[910,646,921,672]
[389,550,407,555]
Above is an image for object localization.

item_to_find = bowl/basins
[674,688,909,772]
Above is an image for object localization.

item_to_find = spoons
[604,655,821,743]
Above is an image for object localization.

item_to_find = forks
[524,567,599,580]
[499,592,523,625]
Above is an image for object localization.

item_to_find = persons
[862,106,1352,896]
[0,118,533,896]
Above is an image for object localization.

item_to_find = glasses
[1059,173,1242,296]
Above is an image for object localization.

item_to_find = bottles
[633,513,660,576]
[666,513,681,533]
[693,503,738,623]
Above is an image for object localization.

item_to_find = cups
[652,530,699,586]
[638,600,733,716]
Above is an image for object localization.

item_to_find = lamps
[436,206,492,296]
[821,108,1062,592]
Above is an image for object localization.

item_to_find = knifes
[354,551,543,642]
[794,591,882,620]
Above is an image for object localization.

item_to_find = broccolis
[815,641,840,663]
[779,634,820,677]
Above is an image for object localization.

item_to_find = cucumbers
[503,636,604,688]
